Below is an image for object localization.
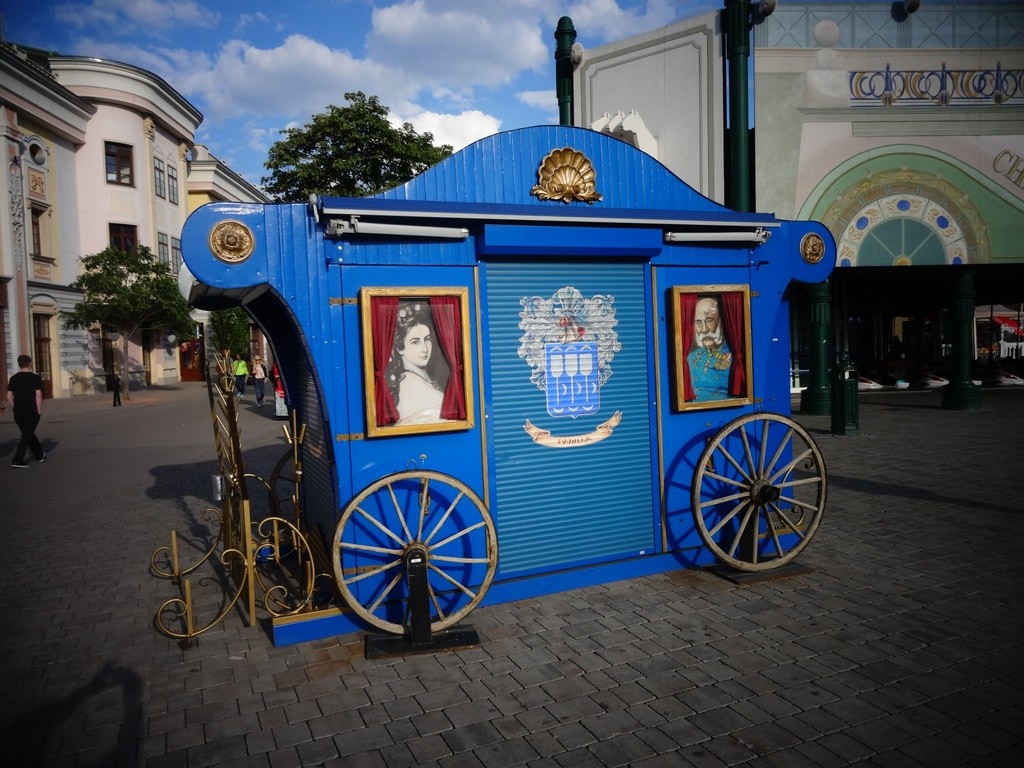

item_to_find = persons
[893,336,901,348]
[219,352,233,398]
[232,354,248,397]
[249,355,269,407]
[6,354,46,467]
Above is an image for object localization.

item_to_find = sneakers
[11,458,29,467]
[236,392,244,398]
[37,451,46,463]
[258,401,263,408]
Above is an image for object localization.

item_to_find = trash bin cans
[830,351,860,437]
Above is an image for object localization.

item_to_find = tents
[971,304,1024,360]
